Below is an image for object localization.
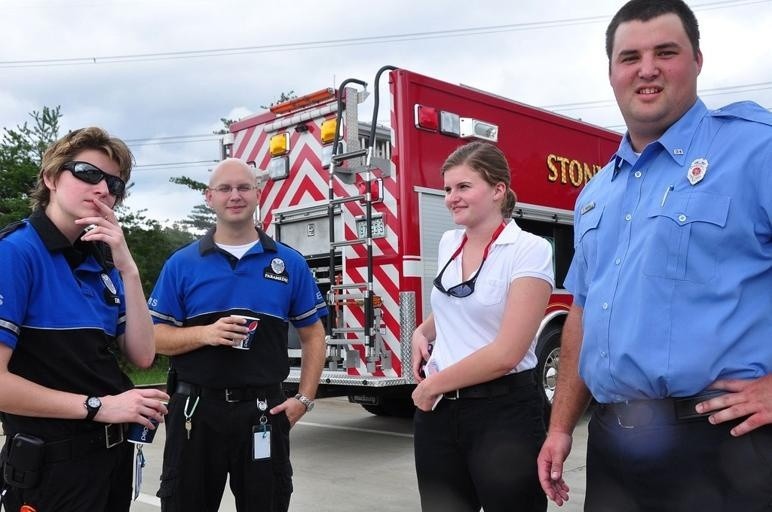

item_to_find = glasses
[209,184,257,195]
[58,160,125,194]
[433,257,486,297]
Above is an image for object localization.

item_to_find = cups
[126,399,169,445]
[229,315,262,351]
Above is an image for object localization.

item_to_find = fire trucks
[220,65,623,416]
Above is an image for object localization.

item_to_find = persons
[408,137,563,510]
[1,126,172,511]
[535,1,771,511]
[145,155,329,511]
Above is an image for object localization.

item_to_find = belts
[436,369,539,401]
[174,381,284,403]
[8,422,132,449]
[598,391,733,428]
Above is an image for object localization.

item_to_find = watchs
[83,394,102,422]
[294,392,315,413]
